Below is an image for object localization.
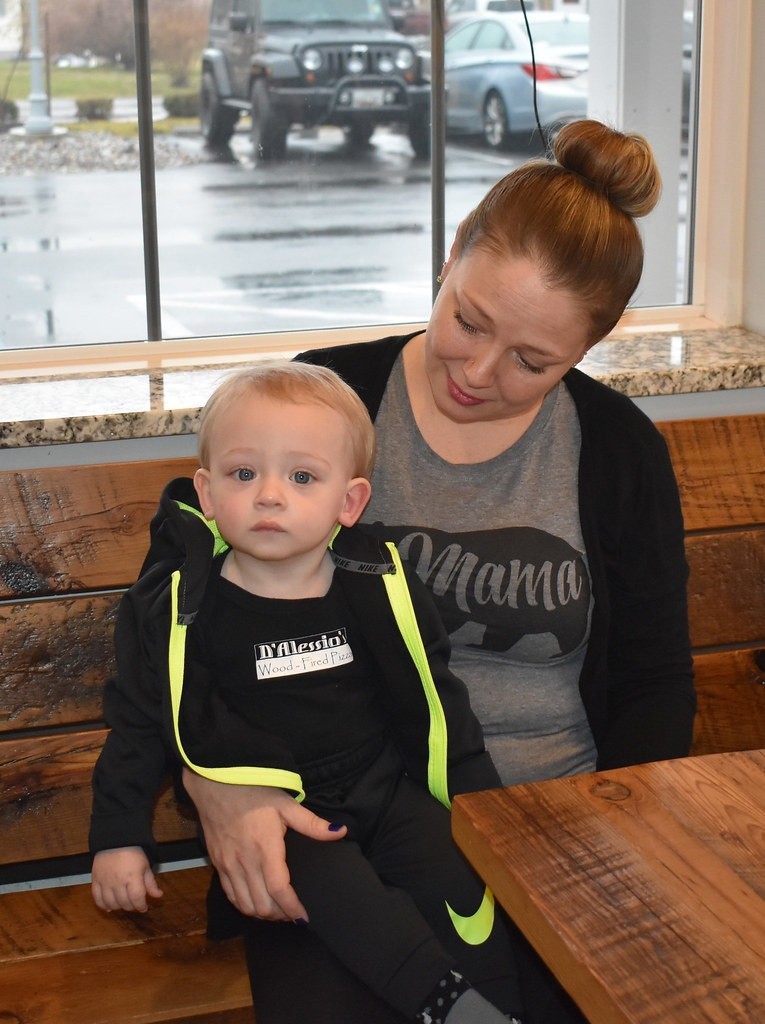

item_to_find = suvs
[199,0,430,160]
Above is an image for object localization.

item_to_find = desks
[453,748,765,1024]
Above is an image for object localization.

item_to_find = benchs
[0,415,765,1024]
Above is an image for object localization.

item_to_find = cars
[443,13,587,149]
[679,10,698,118]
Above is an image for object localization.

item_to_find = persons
[90,361,587,1024]
[114,120,698,1024]
